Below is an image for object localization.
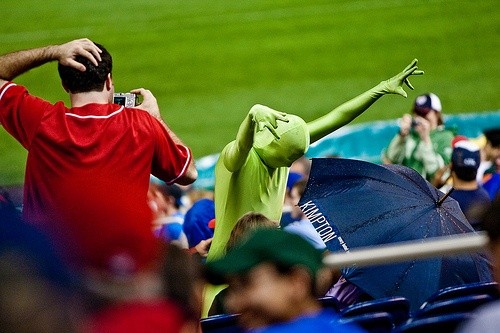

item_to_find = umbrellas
[295,157,492,310]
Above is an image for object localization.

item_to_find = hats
[200,225,324,285]
[452,137,480,175]
[414,92,444,125]
[482,126,500,147]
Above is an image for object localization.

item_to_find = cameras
[113,93,136,108]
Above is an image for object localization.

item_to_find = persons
[0,58,499,333]
[0,39,198,308]
